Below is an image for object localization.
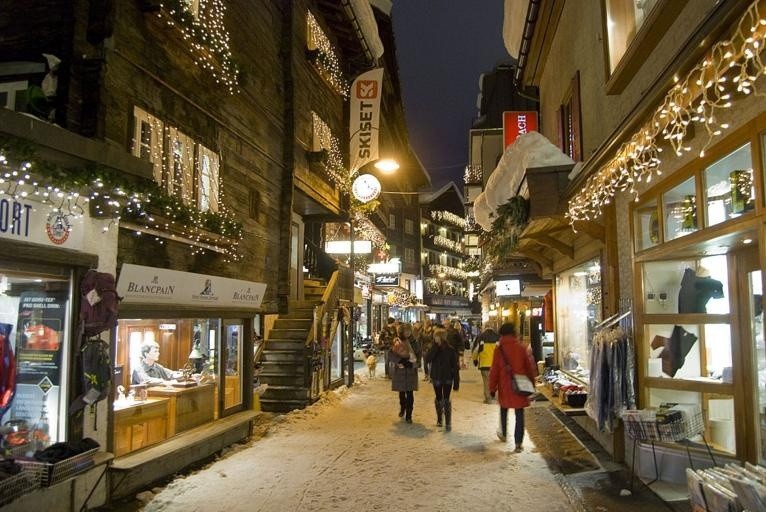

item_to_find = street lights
[348,127,400,389]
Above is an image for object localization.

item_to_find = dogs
[365,355,377,380]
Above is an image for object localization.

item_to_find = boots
[434,399,453,431]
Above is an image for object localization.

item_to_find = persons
[471,320,497,404]
[486,322,538,452]
[378,317,483,386]
[424,327,461,432]
[385,323,423,425]
[131,340,183,386]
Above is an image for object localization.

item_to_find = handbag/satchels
[511,374,535,397]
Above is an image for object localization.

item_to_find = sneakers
[399,411,413,423]
[496,431,507,442]
[515,446,524,453]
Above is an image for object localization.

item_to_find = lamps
[188,338,213,360]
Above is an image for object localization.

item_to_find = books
[681,460,766,511]
[621,401,706,443]
